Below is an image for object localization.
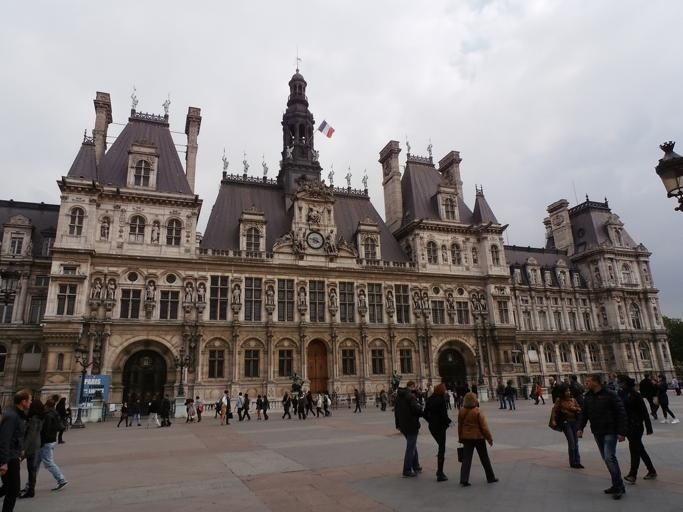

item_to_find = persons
[256,395,269,420]
[329,289,394,308]
[232,286,306,306]
[118,391,172,427]
[93,280,115,300]
[214,390,251,425]
[471,295,486,311]
[184,284,205,302]
[448,295,455,308]
[379,389,388,411]
[541,365,680,499]
[184,395,203,423]
[354,389,362,413]
[456,392,498,484]
[394,381,424,476]
[280,392,332,419]
[147,283,156,301]
[441,246,446,263]
[389,383,420,406]
[422,383,451,481]
[530,381,544,404]
[412,293,428,309]
[152,223,159,241]
[497,382,517,411]
[0,388,69,511]
[423,381,478,408]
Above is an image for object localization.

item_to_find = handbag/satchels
[457,447,463,462]
[548,411,567,432]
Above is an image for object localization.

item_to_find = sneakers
[403,472,417,477]
[487,478,498,483]
[436,471,448,481]
[462,481,471,486]
[661,420,669,424]
[604,485,625,500]
[643,473,657,479]
[415,468,423,472]
[671,419,680,424]
[19,483,35,498]
[624,475,636,483]
[51,481,68,492]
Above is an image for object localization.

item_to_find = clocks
[306,231,324,249]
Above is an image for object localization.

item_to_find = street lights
[173,345,192,419]
[71,342,103,431]
[655,140,683,213]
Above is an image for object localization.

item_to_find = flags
[318,121,334,139]
